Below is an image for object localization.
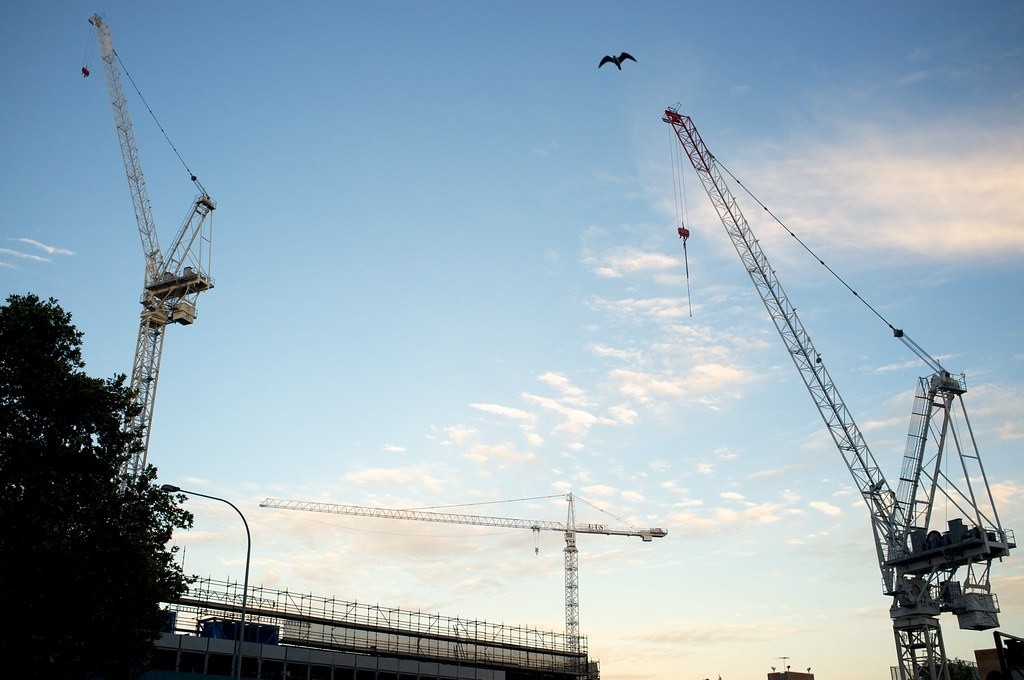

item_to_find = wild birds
[598,50,637,72]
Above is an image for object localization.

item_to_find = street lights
[161,484,251,680]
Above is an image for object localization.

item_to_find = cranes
[83,11,216,498]
[663,101,1017,680]
[260,491,668,680]
